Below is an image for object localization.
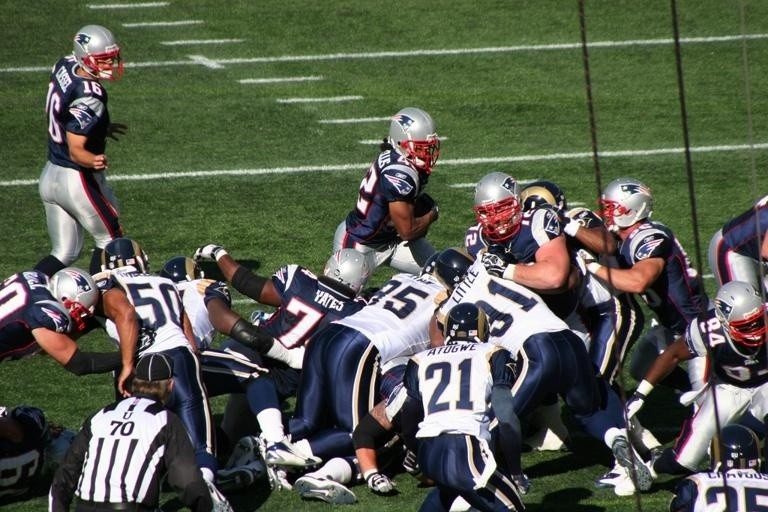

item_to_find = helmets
[443,302,491,343]
[50,266,98,330]
[387,107,440,174]
[473,172,523,243]
[714,281,767,348]
[521,181,568,213]
[596,177,654,232]
[160,256,203,280]
[708,424,762,472]
[420,247,475,292]
[104,238,149,273]
[41,421,79,477]
[322,248,369,296]
[73,25,123,81]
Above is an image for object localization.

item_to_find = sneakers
[205,433,324,511]
[596,435,665,495]
[295,472,358,504]
[250,309,266,326]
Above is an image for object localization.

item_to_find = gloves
[623,379,654,431]
[285,345,306,370]
[194,244,228,263]
[482,252,516,281]
[511,470,530,495]
[363,467,397,493]
[574,248,601,274]
[135,326,157,354]
[540,202,581,237]
[402,449,423,478]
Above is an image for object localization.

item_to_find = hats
[136,353,178,379]
